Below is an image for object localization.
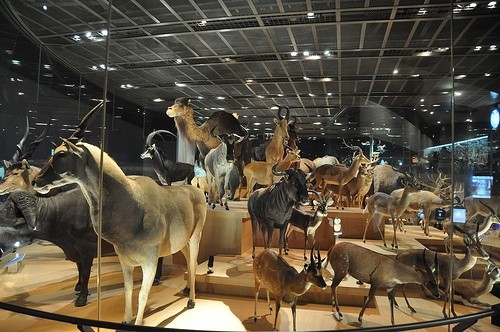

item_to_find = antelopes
[234,104,455,248]
[138,127,196,186]
[448,259,500,309]
[33,98,207,326]
[0,191,164,308]
[392,218,489,320]
[247,163,313,259]
[251,242,328,332]
[455,195,500,224]
[1,115,79,194]
[201,120,251,211]
[443,200,500,256]
[325,240,441,326]
[283,190,333,261]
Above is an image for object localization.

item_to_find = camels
[166,96,251,201]
